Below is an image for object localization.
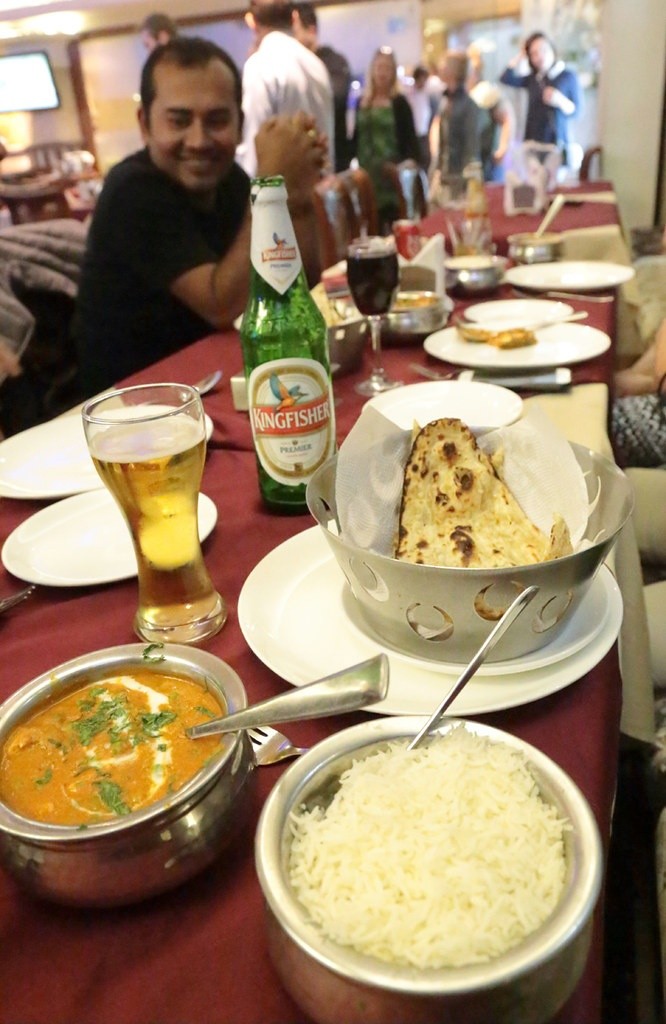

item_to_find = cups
[442,196,491,253]
[83,384,232,644]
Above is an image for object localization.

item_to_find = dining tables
[2,176,638,1024]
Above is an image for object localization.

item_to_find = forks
[0,582,35,613]
[246,722,309,768]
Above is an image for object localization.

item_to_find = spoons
[185,653,389,742]
[534,195,566,235]
[191,369,224,394]
[407,585,543,750]
[465,311,590,350]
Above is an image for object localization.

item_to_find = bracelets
[656,373,665,399]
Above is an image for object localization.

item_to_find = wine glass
[346,236,407,396]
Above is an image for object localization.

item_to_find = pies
[388,416,577,618]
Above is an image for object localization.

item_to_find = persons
[233,1,336,185]
[290,1,350,173]
[613,315,666,467]
[139,14,178,52]
[613,469,666,693]
[402,47,517,184]
[501,32,579,166]
[350,46,422,222]
[70,36,330,397]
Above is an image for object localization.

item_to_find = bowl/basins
[468,299,574,343]
[255,714,605,1023]
[445,256,509,290]
[0,644,261,913]
[505,232,566,265]
[377,290,455,343]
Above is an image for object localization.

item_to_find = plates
[503,259,637,294]
[1,486,219,588]
[422,322,613,369]
[237,517,624,717]
[0,405,213,500]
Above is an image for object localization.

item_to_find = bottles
[241,175,338,518]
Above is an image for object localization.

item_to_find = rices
[294,718,576,976]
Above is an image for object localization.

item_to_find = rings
[307,128,317,145]
